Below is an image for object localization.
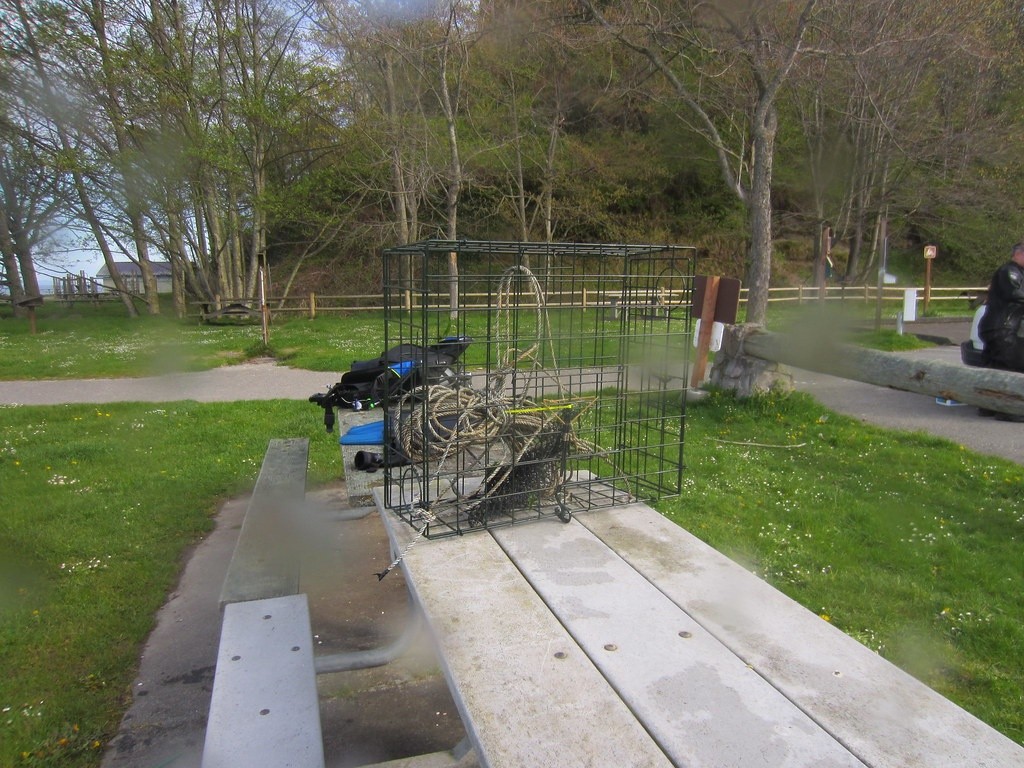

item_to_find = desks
[373,470,1024,767]
[338,408,515,507]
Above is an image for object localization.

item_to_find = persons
[970,243,1024,422]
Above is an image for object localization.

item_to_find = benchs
[203,434,480,768]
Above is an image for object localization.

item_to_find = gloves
[354,451,387,472]
